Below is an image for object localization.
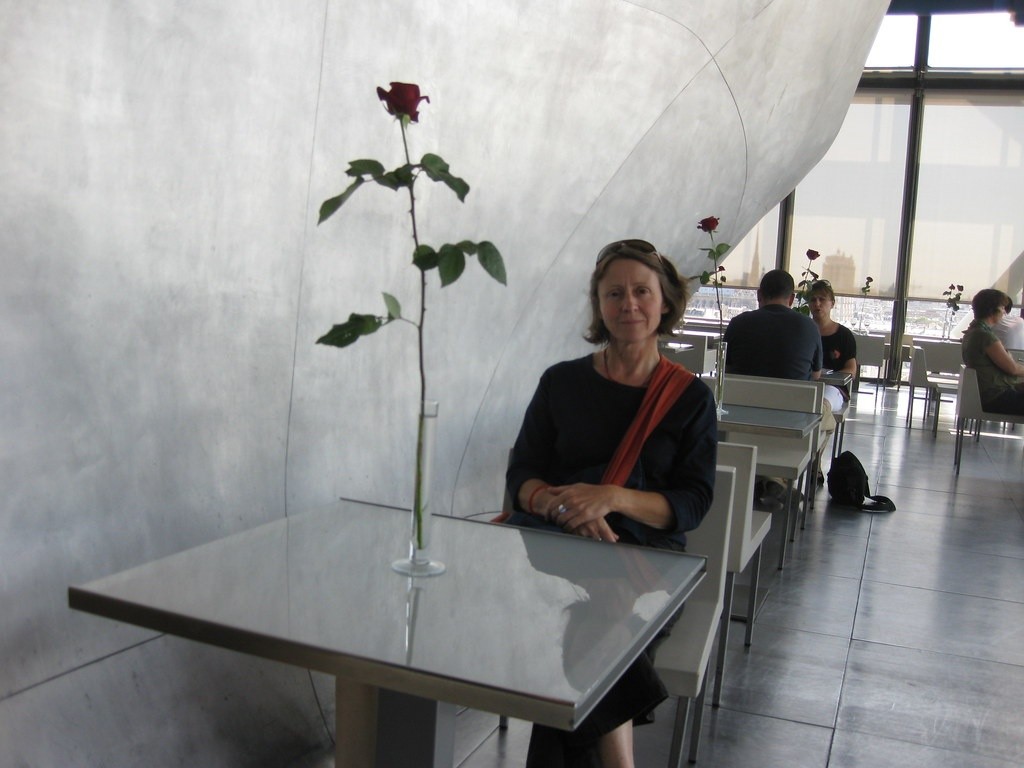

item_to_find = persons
[961,289,1024,415]
[807,279,857,483]
[722,268,837,506]
[502,238,718,768]
[991,296,1024,350]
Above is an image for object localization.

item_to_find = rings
[559,504,566,513]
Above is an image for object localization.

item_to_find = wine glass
[864,320,870,334]
[851,318,857,331]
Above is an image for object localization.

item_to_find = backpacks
[827,451,897,511]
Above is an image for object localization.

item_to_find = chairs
[500,314,1024,768]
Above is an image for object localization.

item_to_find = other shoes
[776,484,804,506]
[755,498,764,504]
[811,469,824,485]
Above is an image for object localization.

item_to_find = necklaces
[604,346,613,381]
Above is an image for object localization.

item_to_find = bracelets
[528,485,549,515]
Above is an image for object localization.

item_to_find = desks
[668,342,694,350]
[672,329,725,340]
[913,337,964,409]
[65,496,714,768]
[714,403,823,626]
[803,372,853,502]
[851,333,886,392]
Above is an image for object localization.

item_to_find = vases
[404,576,427,666]
[393,399,447,577]
[713,342,729,417]
[946,315,954,343]
[939,315,948,343]
[850,304,869,335]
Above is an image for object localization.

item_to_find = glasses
[812,279,831,288]
[596,239,663,269]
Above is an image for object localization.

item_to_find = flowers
[942,284,964,339]
[696,215,731,405]
[858,276,874,333]
[791,248,821,317]
[314,83,509,550]
